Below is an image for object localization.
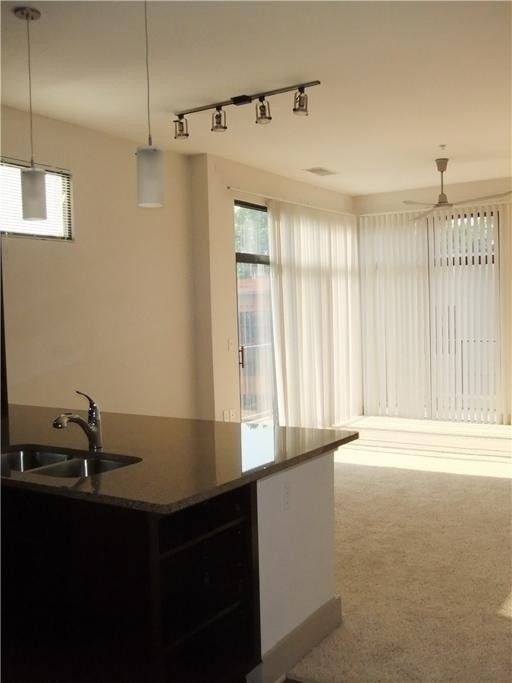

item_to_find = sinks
[32,452,143,478]
[1,444,73,472]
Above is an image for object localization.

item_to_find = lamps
[174,81,320,139]
[136,0,164,207]
[12,5,47,220]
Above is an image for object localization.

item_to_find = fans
[402,157,504,223]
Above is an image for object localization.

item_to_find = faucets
[52,389,103,450]
